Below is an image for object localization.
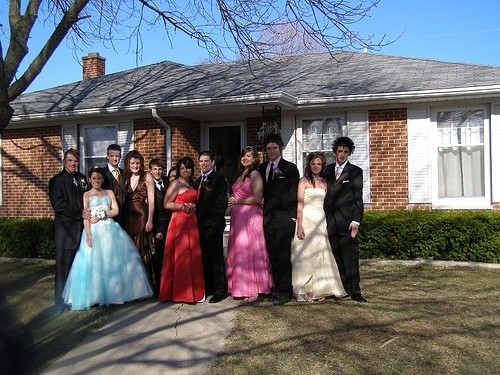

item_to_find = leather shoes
[210,294,229,303]
[351,294,367,302]
[273,295,292,305]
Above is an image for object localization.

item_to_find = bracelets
[181,203,190,212]
[235,197,238,204]
[95,209,106,219]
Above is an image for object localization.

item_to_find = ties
[200,176,206,191]
[336,166,341,181]
[113,169,121,183]
[158,181,163,191]
[268,163,275,182]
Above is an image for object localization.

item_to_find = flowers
[182,202,193,213]
[95,210,106,219]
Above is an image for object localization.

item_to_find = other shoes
[319,297,325,301]
[304,299,316,304]
[248,293,259,303]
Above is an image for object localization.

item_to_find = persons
[257,134,301,306]
[159,156,206,303]
[48,147,91,311]
[167,166,177,184]
[102,143,127,234]
[146,158,172,297]
[290,151,349,303]
[225,145,278,303]
[122,149,156,289]
[61,166,156,310]
[322,136,369,303]
[193,148,229,303]
